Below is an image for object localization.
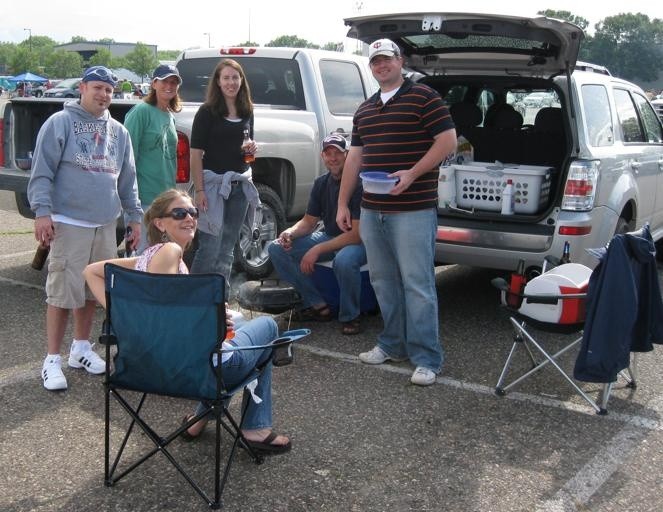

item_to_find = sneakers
[411,367,437,385]
[41,359,68,390]
[68,342,111,374]
[359,345,409,364]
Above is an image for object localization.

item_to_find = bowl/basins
[359,171,399,195]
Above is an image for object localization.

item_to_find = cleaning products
[502,179,515,214]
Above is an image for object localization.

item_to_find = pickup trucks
[1,45,378,279]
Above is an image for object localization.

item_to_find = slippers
[182,414,195,441]
[237,432,291,450]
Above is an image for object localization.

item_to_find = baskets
[451,161,552,213]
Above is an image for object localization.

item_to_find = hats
[153,64,182,84]
[323,135,346,152]
[83,65,117,87]
[368,38,400,63]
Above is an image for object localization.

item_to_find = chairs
[490,221,650,415]
[449,101,567,164]
[104,262,311,510]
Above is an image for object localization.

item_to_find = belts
[230,180,243,184]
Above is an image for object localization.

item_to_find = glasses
[82,68,118,83]
[159,208,199,219]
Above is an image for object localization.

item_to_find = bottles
[226,328,236,340]
[242,130,255,164]
[508,257,526,306]
[560,241,571,264]
[118,226,138,258]
[500,179,515,215]
[31,240,50,271]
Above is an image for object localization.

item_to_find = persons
[646,87,663,97]
[82,189,292,451]
[27,75,54,99]
[122,64,183,257]
[27,63,144,390]
[113,79,144,99]
[336,37,458,386]
[268,134,367,335]
[188,59,257,304]
[1,78,27,98]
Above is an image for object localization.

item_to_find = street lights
[203,31,212,48]
[23,27,32,54]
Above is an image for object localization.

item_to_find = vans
[342,11,661,333]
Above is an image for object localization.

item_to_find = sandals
[286,304,331,322]
[341,317,360,334]
